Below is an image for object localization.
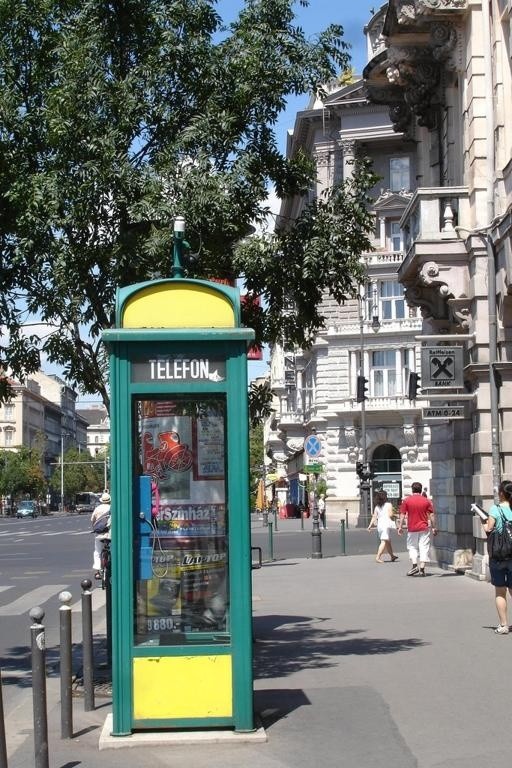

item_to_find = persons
[398,481,437,577]
[316,493,328,530]
[366,491,399,563]
[422,487,428,498]
[476,481,511,635]
[91,492,111,580]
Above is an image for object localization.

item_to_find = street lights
[61,434,70,512]
[357,290,380,515]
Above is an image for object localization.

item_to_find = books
[470,503,491,519]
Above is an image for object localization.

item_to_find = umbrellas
[254,477,266,513]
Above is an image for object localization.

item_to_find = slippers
[95,570,102,580]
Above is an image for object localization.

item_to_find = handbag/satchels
[92,510,111,534]
[387,503,400,530]
[484,504,512,561]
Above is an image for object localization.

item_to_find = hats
[99,493,111,503]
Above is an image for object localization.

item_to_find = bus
[76,492,95,514]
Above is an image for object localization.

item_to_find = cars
[17,501,35,518]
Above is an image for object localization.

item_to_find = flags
[241,295,263,361]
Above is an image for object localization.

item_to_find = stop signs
[304,435,321,458]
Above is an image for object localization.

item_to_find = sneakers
[407,566,419,576]
[418,571,426,577]
[375,558,385,563]
[495,624,509,635]
[391,556,399,562]
